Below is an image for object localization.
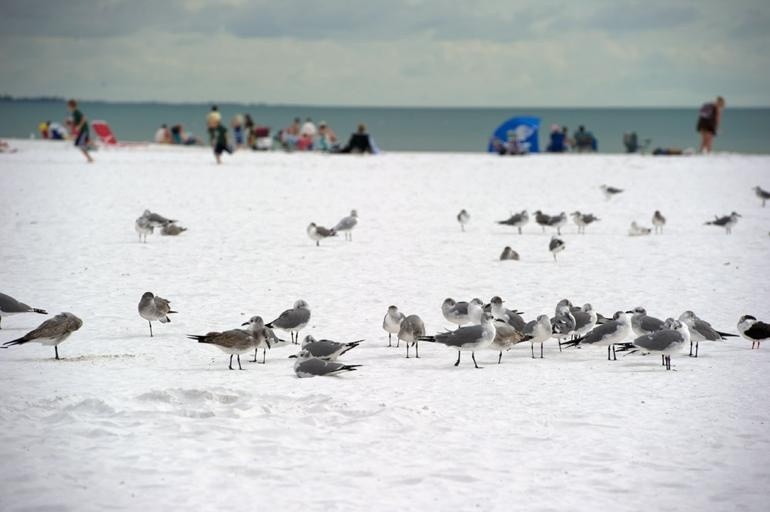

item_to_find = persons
[65,99,100,163]
[695,92,727,154]
[208,105,380,154]
[624,131,693,156]
[154,124,205,145]
[543,125,602,154]
[206,112,233,165]
[37,120,68,140]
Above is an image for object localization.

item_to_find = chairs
[91,118,151,154]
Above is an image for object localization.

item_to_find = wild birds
[457,205,476,233]
[133,208,188,245]
[597,183,627,200]
[138,291,178,338]
[185,298,364,379]
[706,211,742,233]
[382,296,770,372]
[499,205,601,263]
[750,185,769,209]
[330,209,360,241]
[0,293,48,330]
[306,222,336,246]
[2,311,82,360]
[625,210,670,235]
